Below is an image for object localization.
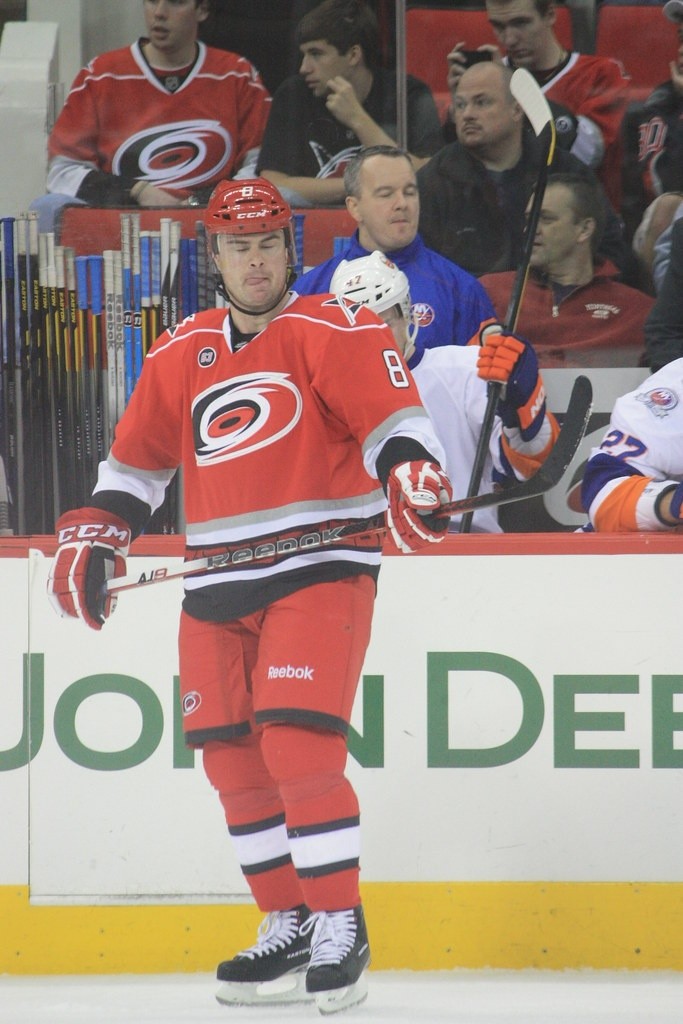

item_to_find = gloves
[385,459,453,555]
[46,507,132,633]
[476,329,547,441]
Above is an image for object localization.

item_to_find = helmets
[203,177,294,257]
[327,251,411,328]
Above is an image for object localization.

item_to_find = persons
[414,60,630,274]
[50,0,272,209]
[445,0,630,225]
[579,355,683,535]
[328,250,563,533]
[48,174,454,1015]
[620,0,682,295]
[289,143,501,346]
[254,0,449,203]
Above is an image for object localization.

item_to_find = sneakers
[306,903,371,1016]
[214,902,315,1005]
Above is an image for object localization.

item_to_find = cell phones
[453,50,492,69]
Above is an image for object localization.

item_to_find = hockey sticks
[0,206,309,539]
[89,375,595,610]
[456,64,560,534]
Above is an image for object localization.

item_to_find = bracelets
[130,179,151,200]
